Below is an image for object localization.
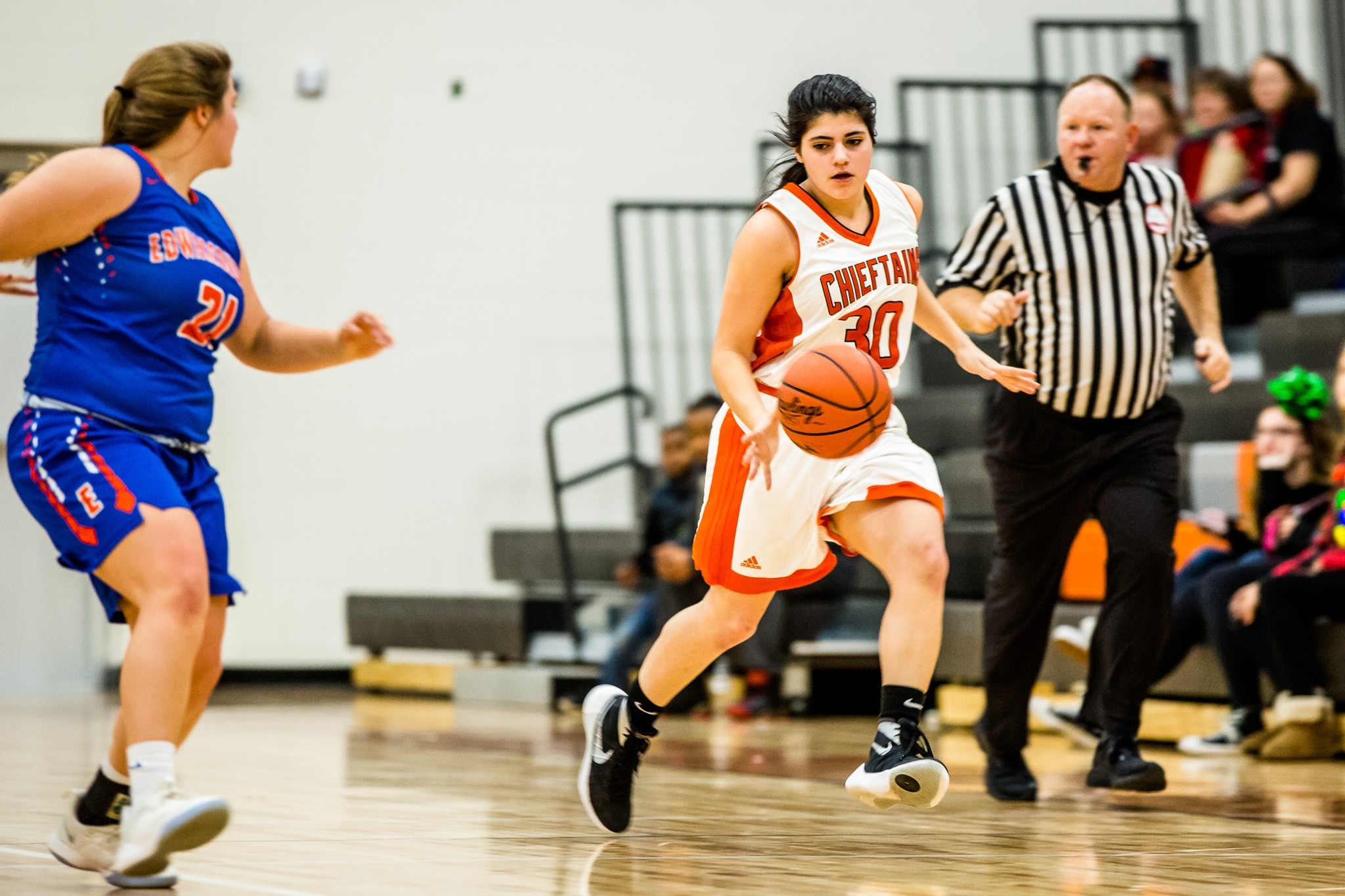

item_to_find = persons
[932,75,1236,804]
[0,43,393,889]
[1027,340,1345,760]
[596,391,859,725]
[1117,53,1345,326]
[576,75,1044,838]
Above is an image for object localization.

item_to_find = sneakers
[1176,709,1268,755]
[975,721,1038,803]
[577,683,654,834]
[43,798,178,887]
[1034,697,1101,743]
[111,796,230,877]
[844,722,949,814]
[1086,743,1168,796]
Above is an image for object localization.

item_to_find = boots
[1261,691,1341,759]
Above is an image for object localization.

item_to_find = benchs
[773,308,1345,723]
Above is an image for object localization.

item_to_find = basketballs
[777,344,892,461]
